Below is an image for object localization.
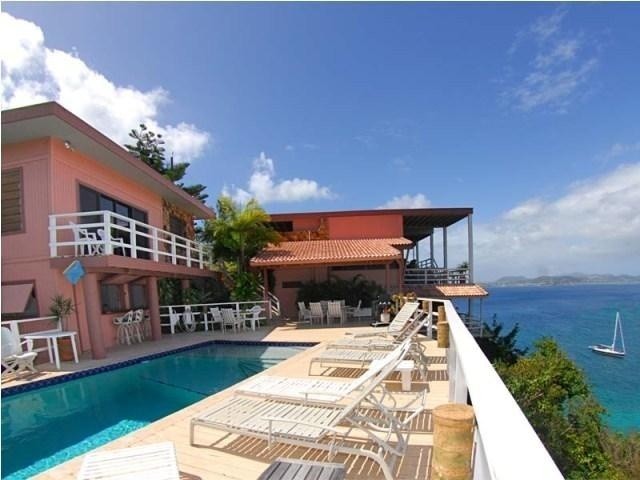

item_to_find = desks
[23,332,79,369]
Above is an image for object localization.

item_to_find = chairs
[96,228,126,257]
[69,222,100,256]
[1,326,38,378]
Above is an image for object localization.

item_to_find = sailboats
[587,310,628,360]
